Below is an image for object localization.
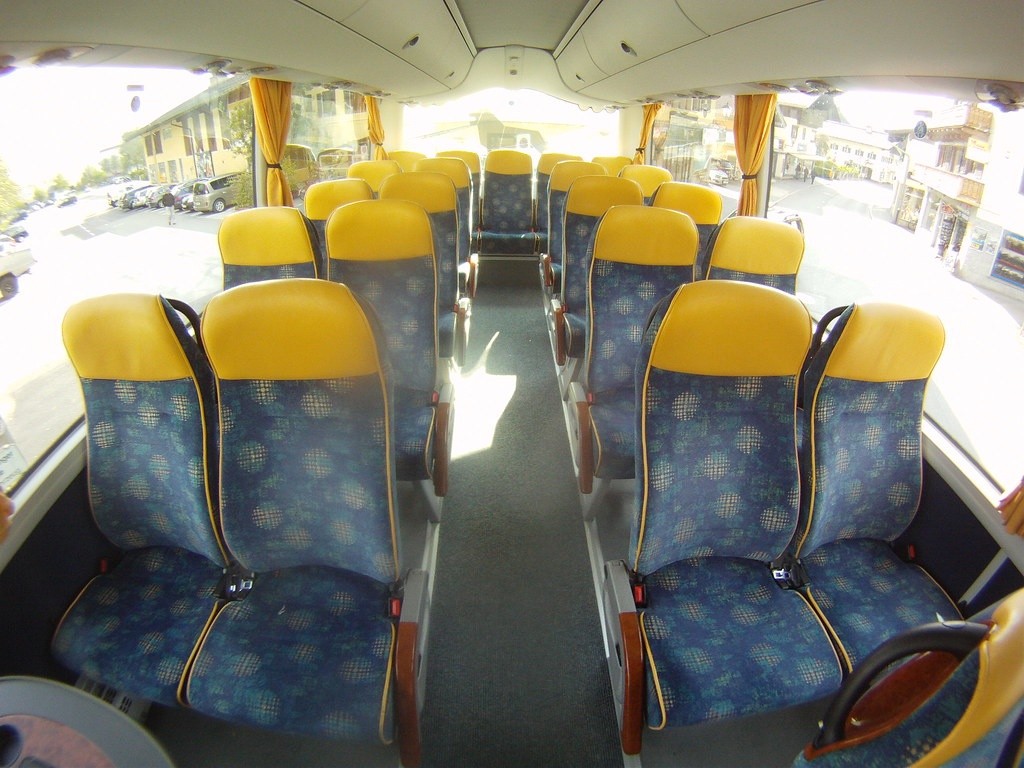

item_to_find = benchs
[62,150,1024,768]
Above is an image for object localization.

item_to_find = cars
[107,171,239,213]
[710,165,728,185]
[0,195,77,300]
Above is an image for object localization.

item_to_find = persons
[162,189,177,225]
[803,167,809,182]
[811,168,818,184]
[794,163,801,178]
[107,193,113,207]
